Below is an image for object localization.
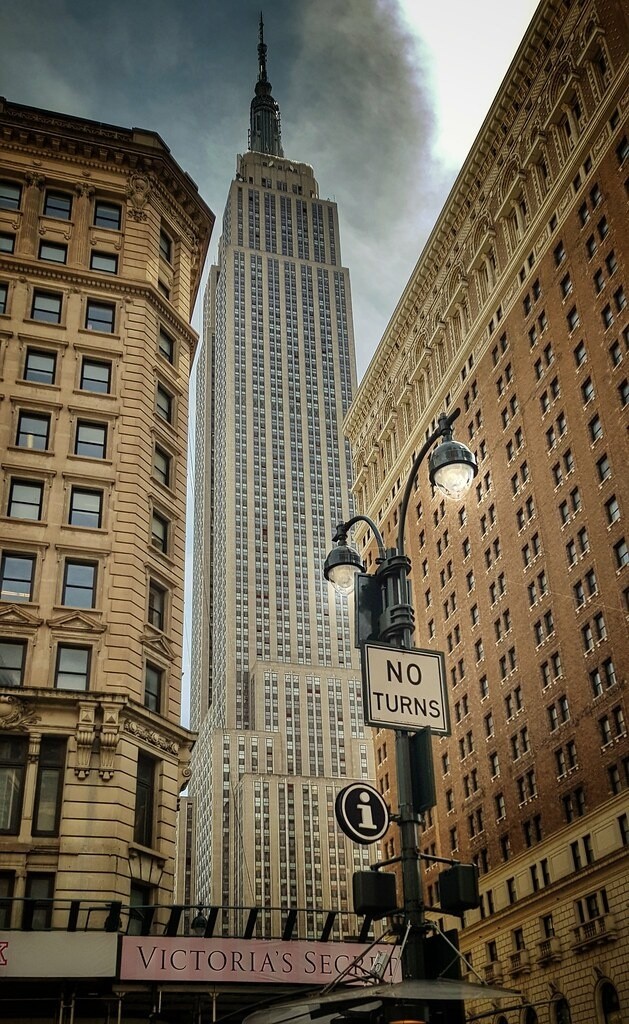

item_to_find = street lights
[323,409,479,981]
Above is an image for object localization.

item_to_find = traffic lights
[354,870,396,916]
[440,863,479,913]
